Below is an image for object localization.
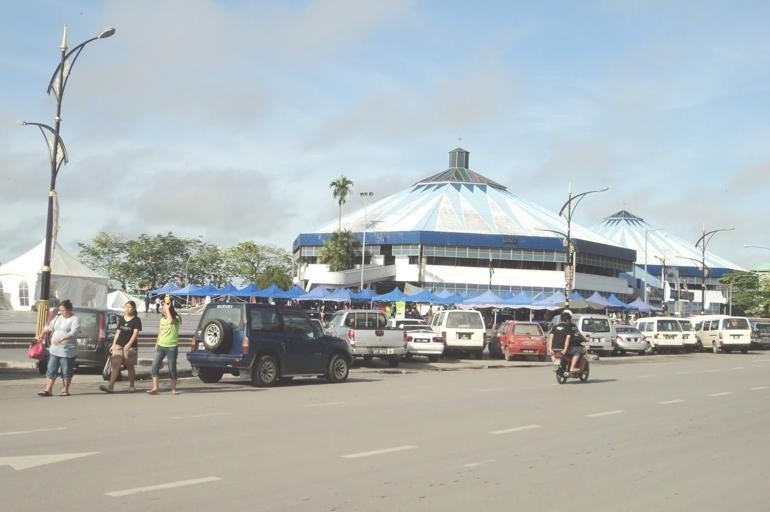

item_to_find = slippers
[37,391,52,396]
[146,389,179,395]
[57,392,71,396]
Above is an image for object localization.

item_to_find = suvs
[185,303,354,386]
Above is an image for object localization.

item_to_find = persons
[99,300,142,392]
[155,296,161,313]
[549,309,587,373]
[144,295,151,312]
[145,303,182,395]
[36,299,80,396]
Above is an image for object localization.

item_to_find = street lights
[185,250,198,288]
[359,191,374,289]
[642,224,770,315]
[528,179,609,314]
[7,23,116,374]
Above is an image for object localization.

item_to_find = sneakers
[100,385,113,392]
[128,387,136,393]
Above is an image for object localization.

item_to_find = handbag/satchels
[27,339,45,359]
[103,352,123,381]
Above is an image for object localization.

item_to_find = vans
[497,318,551,361]
[633,314,770,353]
[548,314,614,355]
[430,307,488,359]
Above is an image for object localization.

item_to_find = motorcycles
[552,335,593,384]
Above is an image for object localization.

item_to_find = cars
[612,325,649,356]
[35,305,139,374]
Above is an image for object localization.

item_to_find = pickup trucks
[388,315,446,362]
[325,308,409,369]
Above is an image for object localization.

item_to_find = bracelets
[60,336,64,341]
